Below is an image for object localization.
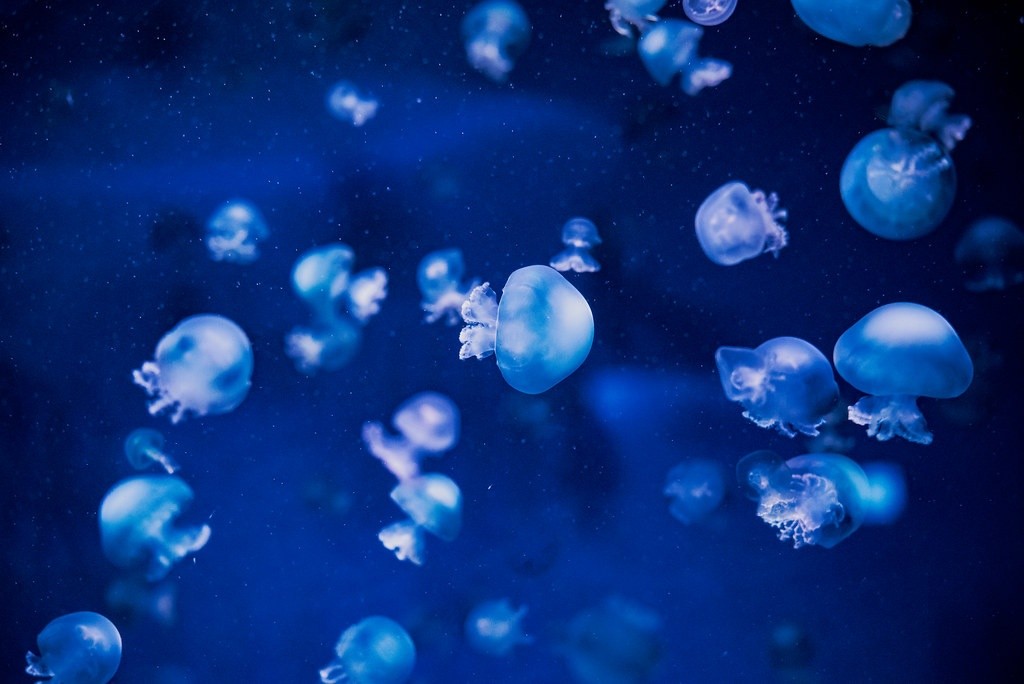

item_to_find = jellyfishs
[25,0,1024,684]
